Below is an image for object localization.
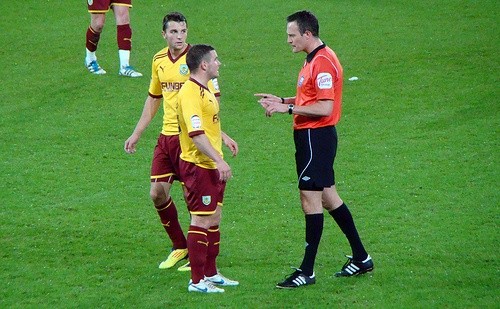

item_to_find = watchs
[288,104,294,115]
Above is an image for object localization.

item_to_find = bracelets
[281,98,284,104]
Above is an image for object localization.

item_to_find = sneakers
[204,272,239,286]
[159,248,188,268]
[119,66,142,77]
[334,254,374,277]
[85,60,106,74]
[276,269,316,289]
[188,278,225,293]
[178,261,191,271]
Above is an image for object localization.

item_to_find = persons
[124,11,221,271]
[175,44,240,292]
[254,11,375,289]
[84,0,143,77]
